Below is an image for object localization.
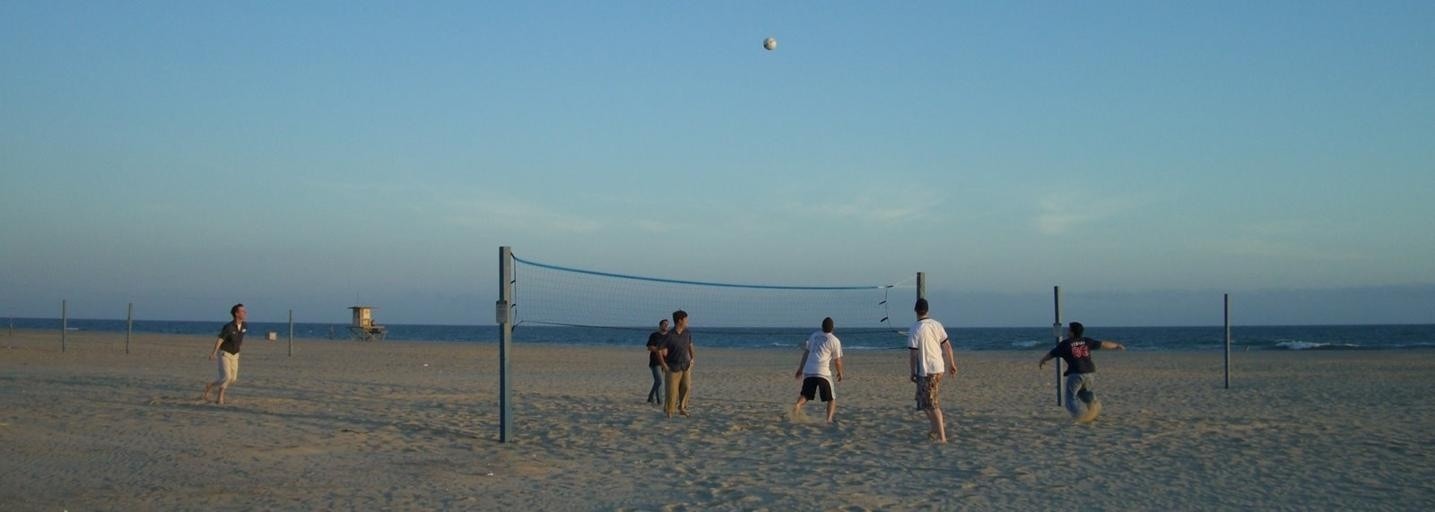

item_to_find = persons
[202,303,247,404]
[905,296,956,442]
[660,308,697,418]
[790,315,843,423]
[646,319,670,408]
[1038,321,1126,424]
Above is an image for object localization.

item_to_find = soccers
[764,38,776,50]
